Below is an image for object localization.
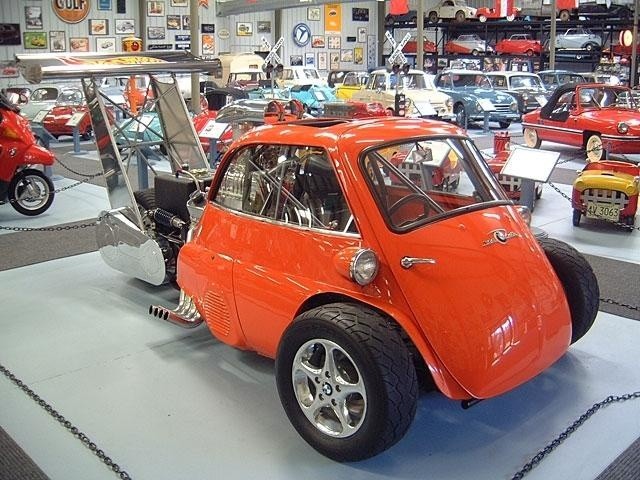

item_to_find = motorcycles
[0,84,58,218]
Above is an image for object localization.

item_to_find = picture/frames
[289,2,376,71]
[0,0,216,56]
[256,20,272,34]
[236,21,254,36]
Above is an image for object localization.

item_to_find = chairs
[288,156,354,232]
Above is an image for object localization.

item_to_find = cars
[0,1,640,163]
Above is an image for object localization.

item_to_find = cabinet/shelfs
[382,19,635,76]
